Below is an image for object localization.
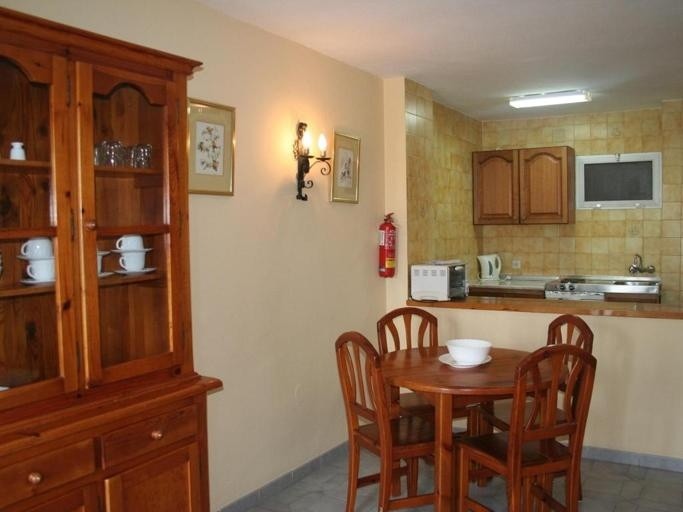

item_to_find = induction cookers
[546,277,615,291]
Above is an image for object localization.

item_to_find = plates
[17,247,157,285]
[438,353,492,369]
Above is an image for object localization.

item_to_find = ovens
[544,291,605,300]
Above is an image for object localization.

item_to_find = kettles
[476,254,501,281]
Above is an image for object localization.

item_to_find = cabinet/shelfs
[0,7,222,512]
[472,146,575,225]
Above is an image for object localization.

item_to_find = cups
[94,140,153,168]
[21,234,146,281]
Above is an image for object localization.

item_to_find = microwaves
[410,259,467,301]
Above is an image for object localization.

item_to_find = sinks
[547,277,615,286]
[610,278,660,286]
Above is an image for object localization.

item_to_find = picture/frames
[330,132,361,203]
[187,94,236,197]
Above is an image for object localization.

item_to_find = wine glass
[10,142,25,160]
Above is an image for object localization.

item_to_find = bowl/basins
[445,339,492,365]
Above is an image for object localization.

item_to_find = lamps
[294,122,333,202]
[507,89,591,109]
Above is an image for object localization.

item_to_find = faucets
[628,253,656,275]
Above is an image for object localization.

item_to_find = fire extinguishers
[377,212,397,279]
